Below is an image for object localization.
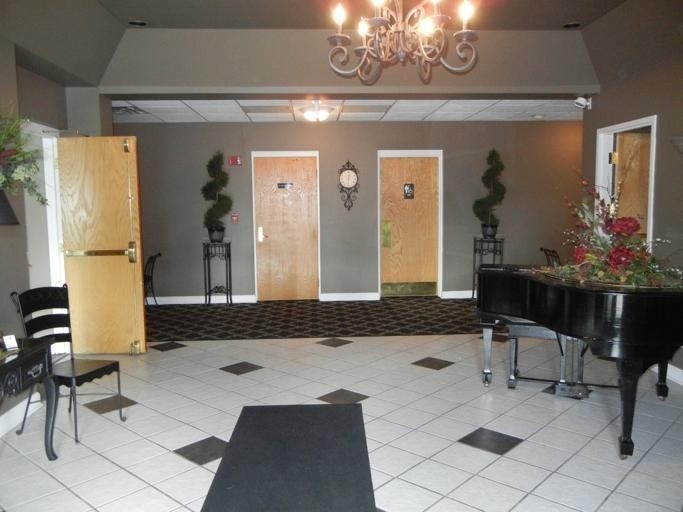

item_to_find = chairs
[143,253,162,313]
[11,283,127,444]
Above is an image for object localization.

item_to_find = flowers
[529,167,682,290]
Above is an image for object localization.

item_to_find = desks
[471,237,504,301]
[0,335,60,463]
[202,239,233,312]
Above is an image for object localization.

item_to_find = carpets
[199,402,377,511]
[143,296,507,342]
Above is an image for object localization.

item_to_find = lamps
[573,95,592,110]
[327,0,480,86]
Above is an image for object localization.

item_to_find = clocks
[336,160,360,211]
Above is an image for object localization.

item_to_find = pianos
[475,263,683,461]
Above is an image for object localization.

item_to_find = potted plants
[199,149,232,243]
[472,148,506,237]
